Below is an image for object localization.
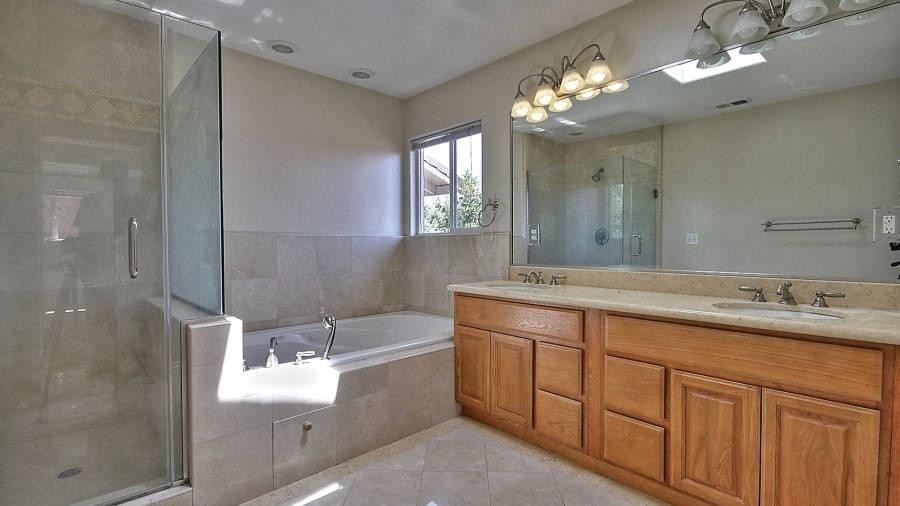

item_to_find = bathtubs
[242,309,457,366]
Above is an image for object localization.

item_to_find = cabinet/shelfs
[451,293,900,506]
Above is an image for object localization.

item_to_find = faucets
[776,279,796,304]
[266,336,281,367]
[526,270,545,284]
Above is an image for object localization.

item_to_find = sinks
[487,283,554,289]
[712,301,844,320]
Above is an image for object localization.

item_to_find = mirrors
[509,0,900,280]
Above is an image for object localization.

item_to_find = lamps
[683,0,883,60]
[525,81,629,122]
[511,44,614,118]
[697,9,880,68]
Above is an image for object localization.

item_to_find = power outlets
[686,233,699,245]
[883,216,896,234]
